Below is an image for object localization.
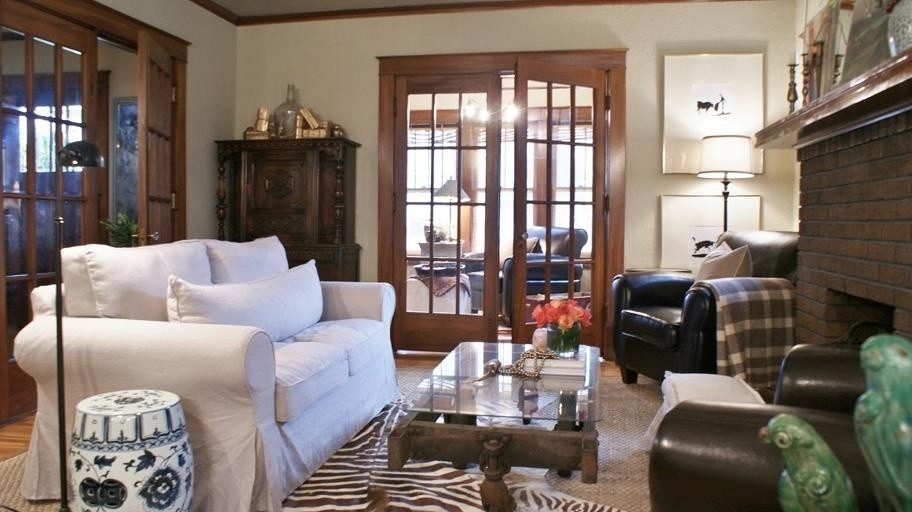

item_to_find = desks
[416,241,463,264]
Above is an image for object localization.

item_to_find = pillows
[166,258,324,342]
[202,234,289,284]
[693,240,753,284]
[63,242,215,321]
[498,236,540,271]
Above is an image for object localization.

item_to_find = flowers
[530,298,593,354]
[885,0,900,13]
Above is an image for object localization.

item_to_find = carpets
[282,395,631,512]
[0,368,664,512]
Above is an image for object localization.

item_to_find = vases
[546,320,581,359]
[886,0,912,58]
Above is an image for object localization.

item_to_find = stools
[67,388,195,512]
[406,272,472,314]
[640,370,767,452]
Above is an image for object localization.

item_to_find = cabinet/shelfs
[214,136,362,282]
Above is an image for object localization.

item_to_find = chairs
[461,225,588,328]
[611,230,800,385]
[648,342,882,512]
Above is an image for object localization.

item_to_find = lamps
[434,176,471,242]
[53,141,106,512]
[695,135,756,232]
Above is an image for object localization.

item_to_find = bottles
[333,124,343,137]
[274,84,303,139]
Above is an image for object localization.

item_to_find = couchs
[12,234,401,512]
